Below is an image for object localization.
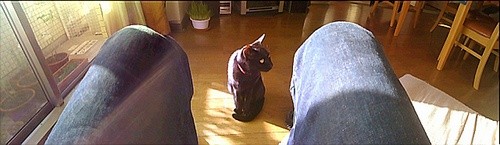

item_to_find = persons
[42,18,431,145]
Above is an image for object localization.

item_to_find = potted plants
[0,77,38,121]
[185,1,213,32]
[52,56,88,93]
[16,71,44,97]
[35,10,69,75]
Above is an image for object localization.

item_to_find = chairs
[429,1,485,42]
[370,0,427,37]
[436,0,499,89]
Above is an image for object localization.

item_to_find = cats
[226,33,274,123]
